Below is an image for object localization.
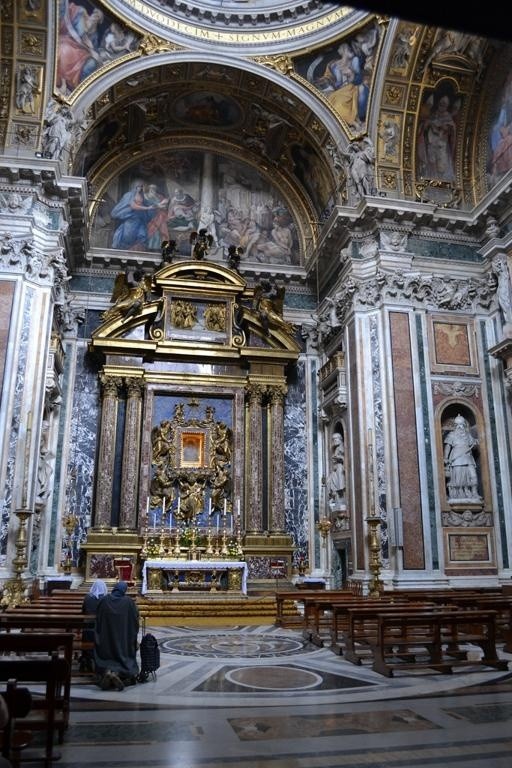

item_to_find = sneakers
[98,673,125,691]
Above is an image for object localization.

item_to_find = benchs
[274,582,511,680]
[0,587,101,759]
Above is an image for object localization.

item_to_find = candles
[146,496,182,531]
[208,497,241,534]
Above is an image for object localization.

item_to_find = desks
[143,560,248,596]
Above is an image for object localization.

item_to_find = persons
[377,117,397,155]
[36,420,57,504]
[416,93,461,181]
[16,65,39,115]
[314,27,377,198]
[486,82,512,175]
[109,178,299,265]
[443,413,484,505]
[0,191,33,216]
[78,579,109,677]
[380,231,408,252]
[40,0,141,160]
[325,431,347,512]
[93,581,139,691]
[391,26,419,69]
